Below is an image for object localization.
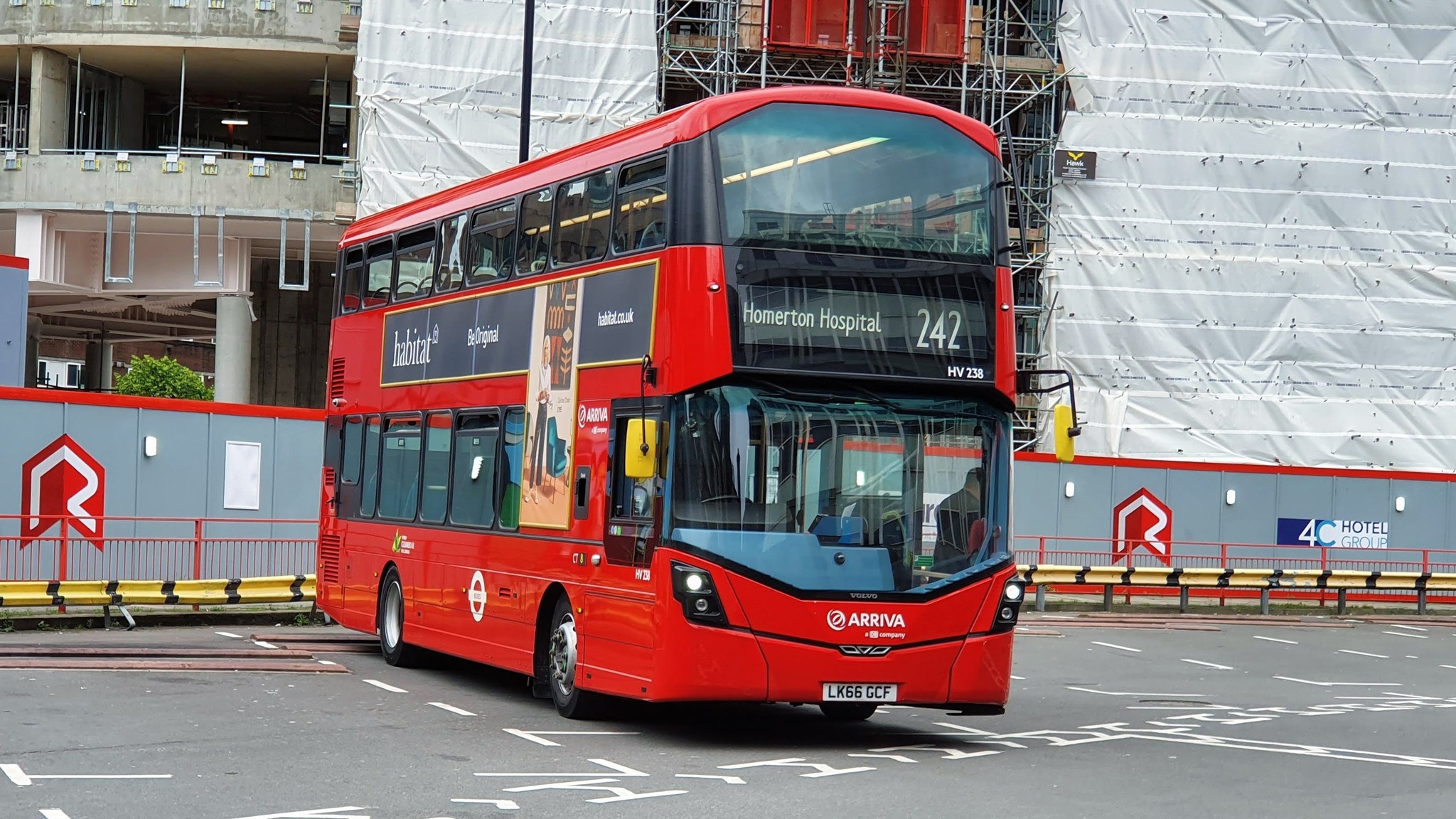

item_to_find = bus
[315,86,1083,721]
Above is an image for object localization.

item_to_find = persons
[933,467,984,575]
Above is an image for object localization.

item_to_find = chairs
[362,469,494,525]
[345,220,669,314]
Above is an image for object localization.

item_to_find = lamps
[221,111,248,125]
